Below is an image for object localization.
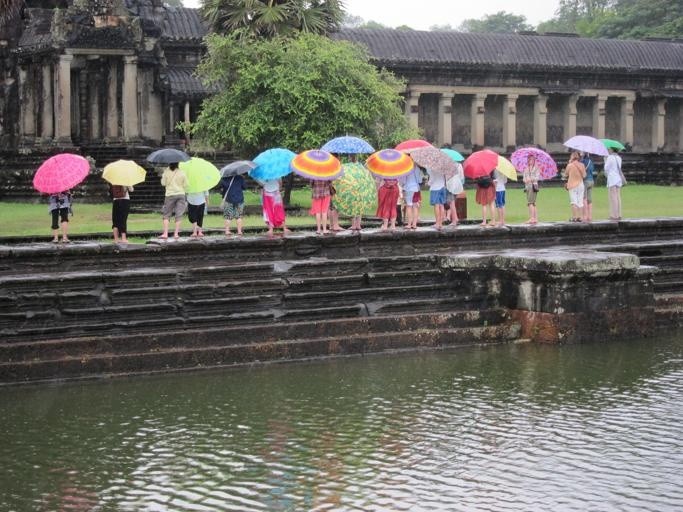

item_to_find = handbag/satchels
[329,181,336,195]
[413,191,421,202]
[533,184,539,192]
[220,201,225,209]
[444,203,450,210]
[478,177,492,188]
[619,168,627,186]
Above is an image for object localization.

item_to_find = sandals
[569,218,581,221]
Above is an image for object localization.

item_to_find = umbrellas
[320,130,376,154]
[393,140,432,150]
[599,138,625,152]
[33,153,90,194]
[563,135,609,157]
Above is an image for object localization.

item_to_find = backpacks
[112,185,127,197]
